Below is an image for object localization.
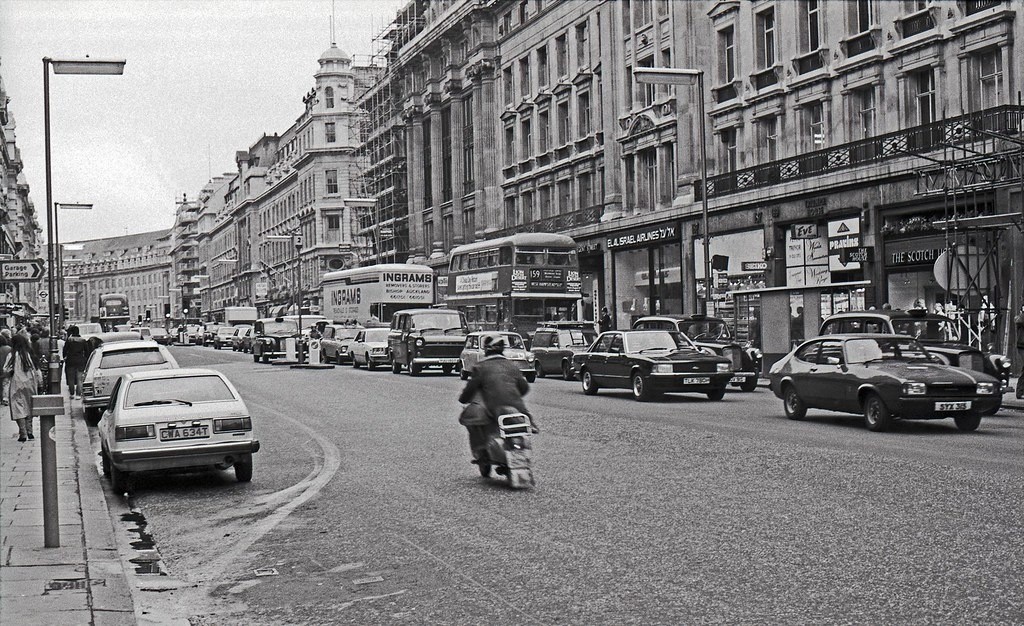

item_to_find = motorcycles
[459,367,535,492]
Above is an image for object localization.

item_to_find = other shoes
[70,394,74,399]
[2,400,9,406]
[27,432,34,439]
[75,395,82,400]
[17,437,26,442]
[471,456,490,466]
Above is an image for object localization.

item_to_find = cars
[766,334,999,432]
[96,369,261,493]
[65,321,172,347]
[458,331,536,383]
[573,328,735,402]
[172,315,390,371]
[81,340,181,425]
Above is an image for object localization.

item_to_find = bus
[445,233,584,342]
[98,293,131,332]
[322,264,435,326]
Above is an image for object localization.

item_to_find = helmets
[482,334,505,356]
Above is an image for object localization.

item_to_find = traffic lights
[63,304,70,320]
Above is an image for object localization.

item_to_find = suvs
[530,321,600,381]
[630,314,763,392]
[817,311,1003,417]
[386,308,471,376]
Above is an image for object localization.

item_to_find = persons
[3,335,38,441]
[747,298,995,347]
[458,335,529,465]
[598,306,610,332]
[0,319,89,406]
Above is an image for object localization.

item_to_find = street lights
[139,259,241,328]
[267,234,304,363]
[40,54,128,395]
[631,66,712,318]
